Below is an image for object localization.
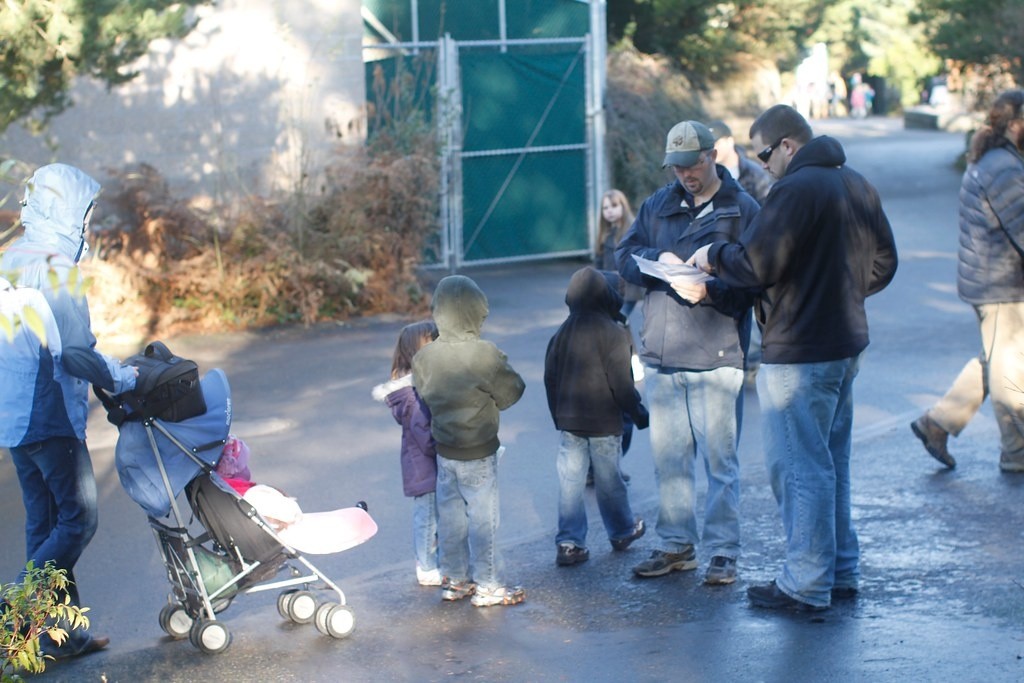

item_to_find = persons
[0,161,140,663]
[583,269,635,489]
[909,89,1024,475]
[612,119,764,589]
[543,266,650,566]
[683,103,901,619]
[592,189,649,383]
[372,321,473,586]
[410,274,528,608]
[698,122,778,394]
[209,433,379,557]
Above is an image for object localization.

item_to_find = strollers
[93,383,356,654]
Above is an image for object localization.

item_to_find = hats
[661,120,716,170]
[215,433,252,477]
[707,120,732,145]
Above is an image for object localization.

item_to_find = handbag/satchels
[91,341,206,425]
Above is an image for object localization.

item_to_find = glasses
[666,150,712,174]
[757,131,791,164]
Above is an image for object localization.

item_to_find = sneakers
[470,581,524,607]
[611,519,645,551]
[556,541,589,565]
[631,545,698,577]
[703,556,737,583]
[441,577,476,601]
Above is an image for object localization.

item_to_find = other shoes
[585,470,632,486]
[911,409,955,467]
[1000,447,1024,473]
[416,564,443,587]
[746,579,857,611]
[356,501,367,512]
[85,637,110,653]
[631,356,644,382]
